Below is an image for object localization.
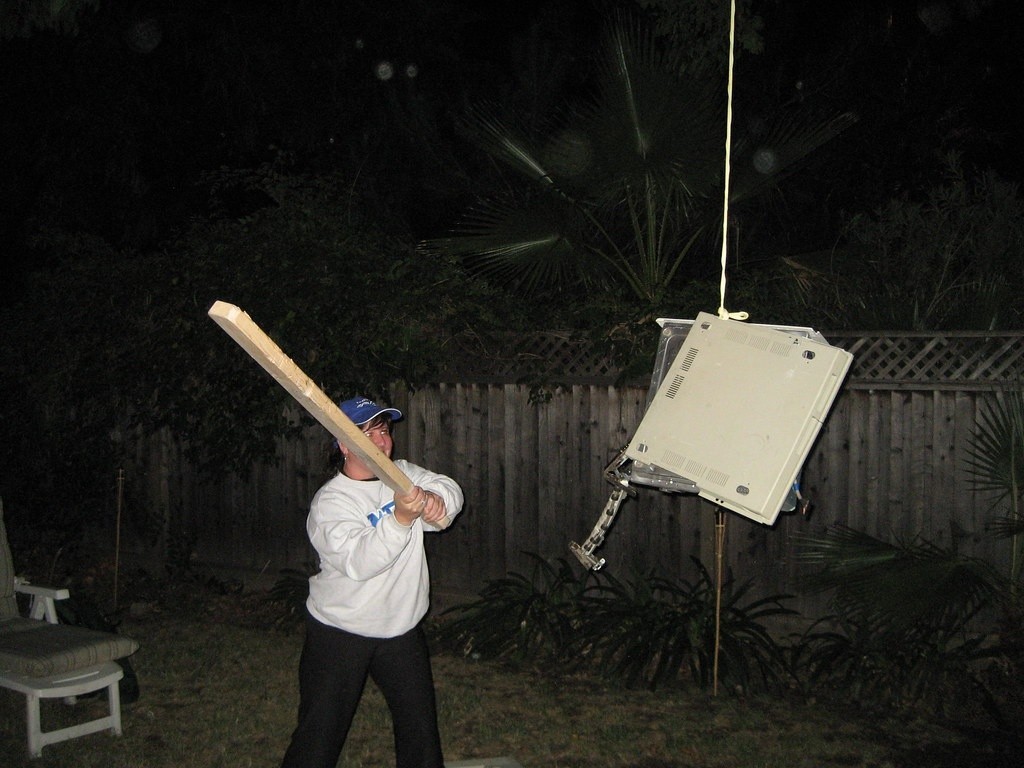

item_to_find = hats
[333,396,403,450]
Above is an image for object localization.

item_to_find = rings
[421,500,426,504]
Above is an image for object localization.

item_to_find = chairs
[0,493,141,762]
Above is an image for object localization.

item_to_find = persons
[281,398,463,768]
